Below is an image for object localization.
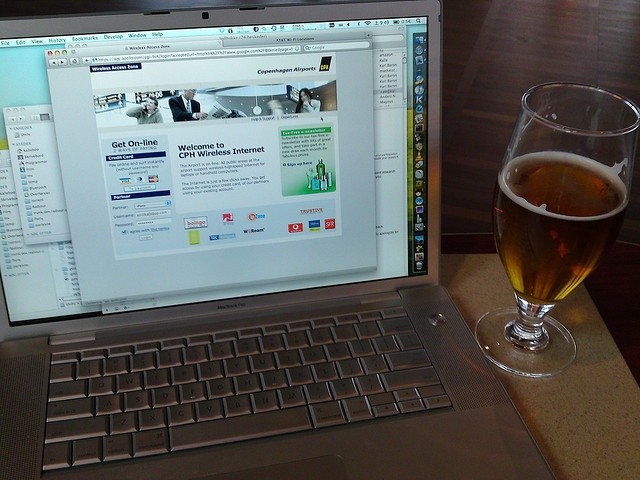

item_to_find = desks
[439,252,640,479]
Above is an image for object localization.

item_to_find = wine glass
[473,81,638,376]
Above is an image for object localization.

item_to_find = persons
[296,88,321,114]
[169,90,208,121]
[125,97,163,125]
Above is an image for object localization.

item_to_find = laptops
[0,0,557,480]
